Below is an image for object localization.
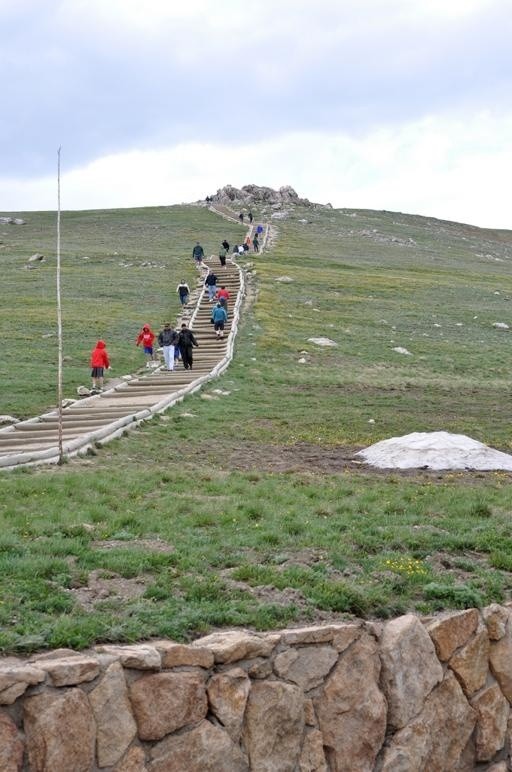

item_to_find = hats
[164,322,170,328]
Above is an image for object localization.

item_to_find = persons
[206,273,230,339]
[89,342,109,390]
[135,322,199,371]
[176,280,190,306]
[191,212,266,265]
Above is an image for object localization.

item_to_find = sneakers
[92,384,104,391]
